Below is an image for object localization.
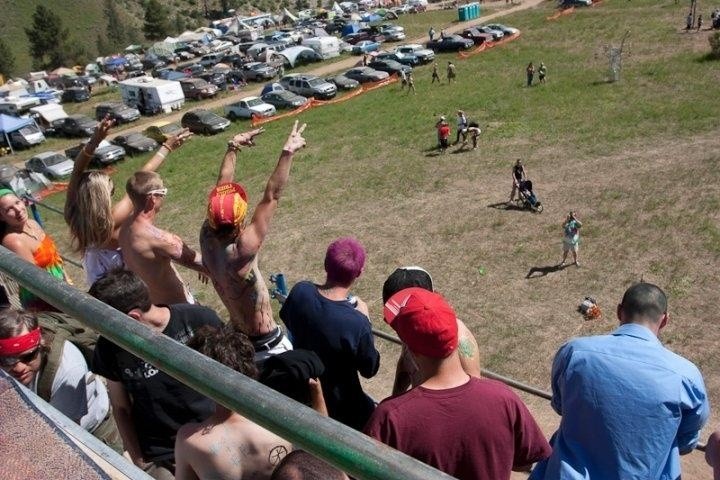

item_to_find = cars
[367,60,412,77]
[119,26,305,81]
[353,41,381,54]
[237,0,426,44]
[181,109,231,136]
[343,67,389,83]
[0,165,33,191]
[0,53,149,125]
[276,73,307,90]
[322,76,359,91]
[338,42,353,54]
[25,152,74,180]
[484,24,518,36]
[141,121,184,145]
[110,133,159,158]
[261,90,308,110]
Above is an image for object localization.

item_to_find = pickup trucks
[224,97,275,122]
[54,115,97,137]
[65,140,125,168]
[426,35,474,54]
[228,62,276,82]
[196,52,224,68]
[457,29,493,44]
[473,26,504,41]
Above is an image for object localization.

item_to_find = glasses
[0,344,43,366]
[146,189,169,196]
[110,188,115,196]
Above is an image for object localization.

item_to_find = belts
[255,331,284,353]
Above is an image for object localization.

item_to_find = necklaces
[16,223,39,240]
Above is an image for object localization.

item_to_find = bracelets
[88,141,99,148]
[81,146,95,159]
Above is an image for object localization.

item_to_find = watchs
[226,140,241,153]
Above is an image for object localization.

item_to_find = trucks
[0,124,43,150]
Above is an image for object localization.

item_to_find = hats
[440,115,445,119]
[383,266,434,303]
[209,183,249,230]
[384,287,458,357]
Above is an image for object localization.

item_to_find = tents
[225,16,251,37]
[281,7,299,24]
[331,1,343,15]
[50,67,76,79]
[341,24,362,37]
[148,27,222,56]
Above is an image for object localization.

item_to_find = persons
[436,116,446,143]
[697,14,702,30]
[400,68,408,88]
[714,14,720,28]
[85,270,225,479]
[432,62,441,83]
[454,109,468,145]
[264,349,351,479]
[428,26,434,42]
[64,113,193,286]
[361,287,533,480]
[439,122,450,153]
[119,171,211,307]
[175,329,292,479]
[686,13,693,29]
[363,55,368,67]
[711,9,718,24]
[407,71,416,93]
[537,62,547,84]
[446,60,457,82]
[384,266,480,396]
[558,211,582,266]
[200,119,306,363]
[439,28,446,42]
[526,62,534,86]
[508,159,528,202]
[462,122,480,148]
[0,189,73,311]
[527,283,710,480]
[0,309,124,456]
[281,239,382,434]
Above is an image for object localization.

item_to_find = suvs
[175,78,218,100]
[96,102,141,124]
[395,44,435,64]
[289,76,336,100]
[371,53,419,69]
